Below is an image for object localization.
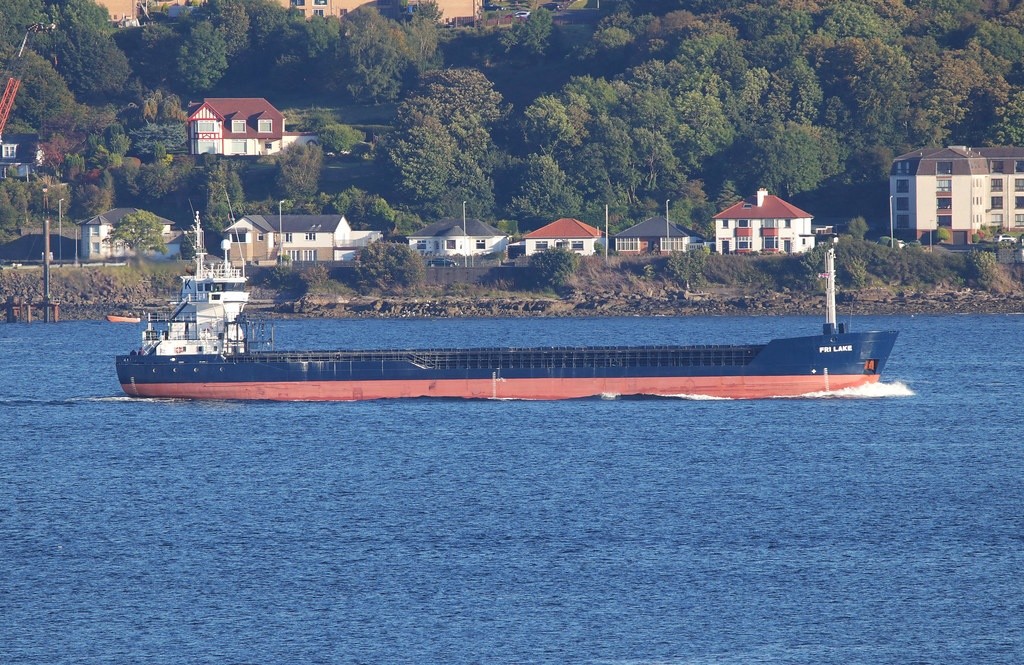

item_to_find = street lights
[666,199,670,256]
[889,195,894,248]
[58,198,64,259]
[280,201,284,266]
[463,201,467,267]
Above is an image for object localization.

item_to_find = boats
[106,315,141,323]
[116,187,899,402]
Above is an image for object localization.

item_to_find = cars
[515,11,530,19]
[538,3,563,11]
[428,258,459,268]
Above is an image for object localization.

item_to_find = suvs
[993,234,1017,243]
